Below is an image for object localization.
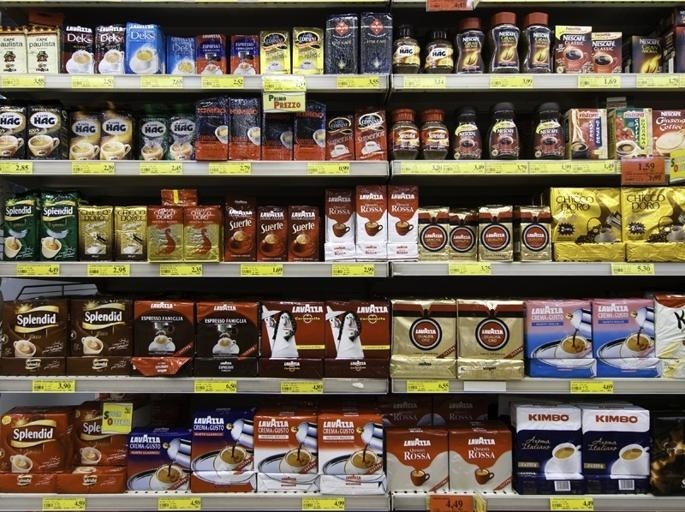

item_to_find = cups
[0,47,684,494]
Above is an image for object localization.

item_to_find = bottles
[455,16,487,74]
[521,12,554,73]
[486,12,522,73]
[423,27,454,73]
[393,23,422,74]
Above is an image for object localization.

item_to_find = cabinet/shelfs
[1,2,685,512]
